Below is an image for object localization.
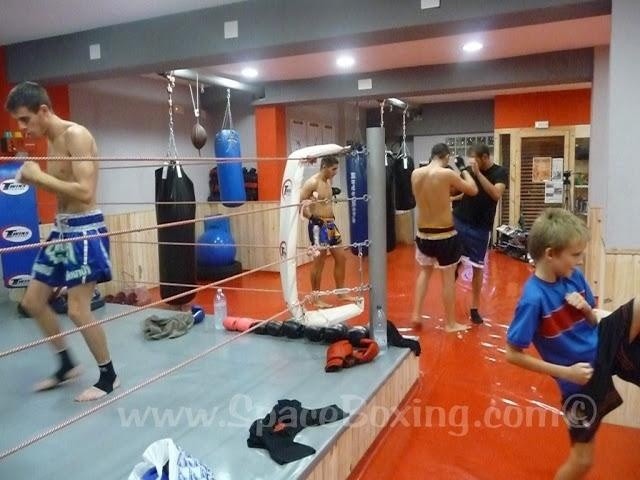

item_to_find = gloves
[332,186,343,195]
[252,319,369,343]
[325,339,353,374]
[453,155,467,172]
[309,215,324,228]
[349,335,380,365]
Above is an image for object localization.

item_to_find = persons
[450,142,510,324]
[5,78,121,403]
[504,205,640,480]
[298,154,358,309]
[409,142,480,333]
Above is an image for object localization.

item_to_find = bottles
[374,305,388,354]
[213,288,228,331]
